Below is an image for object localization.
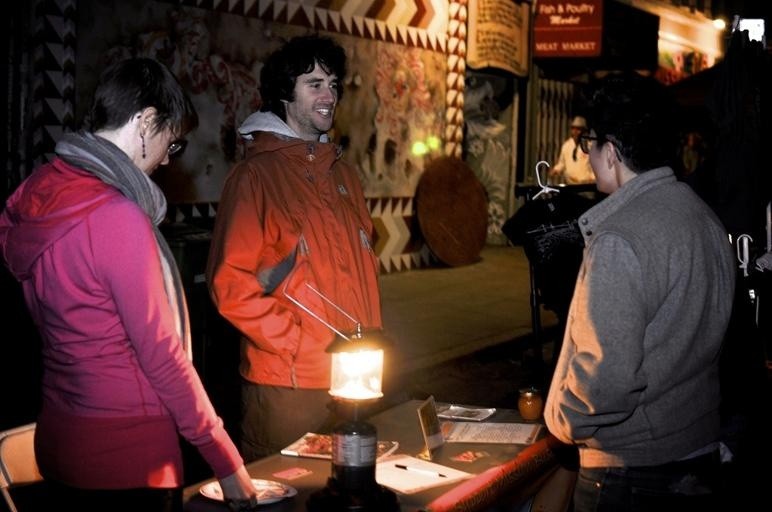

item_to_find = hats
[570,116,587,129]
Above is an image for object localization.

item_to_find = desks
[174,391,569,510]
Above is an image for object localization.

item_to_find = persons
[537,66,736,512]
[2,54,260,512]
[547,111,600,185]
[203,28,383,468]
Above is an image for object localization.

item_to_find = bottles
[518,388,543,422]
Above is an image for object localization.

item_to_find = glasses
[161,118,184,154]
[578,130,623,163]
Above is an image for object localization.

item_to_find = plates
[197,477,299,504]
[280,432,402,466]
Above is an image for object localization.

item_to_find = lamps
[326,325,389,488]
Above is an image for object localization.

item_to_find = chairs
[1,420,49,512]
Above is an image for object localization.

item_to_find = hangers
[735,233,756,277]
[530,159,560,201]
